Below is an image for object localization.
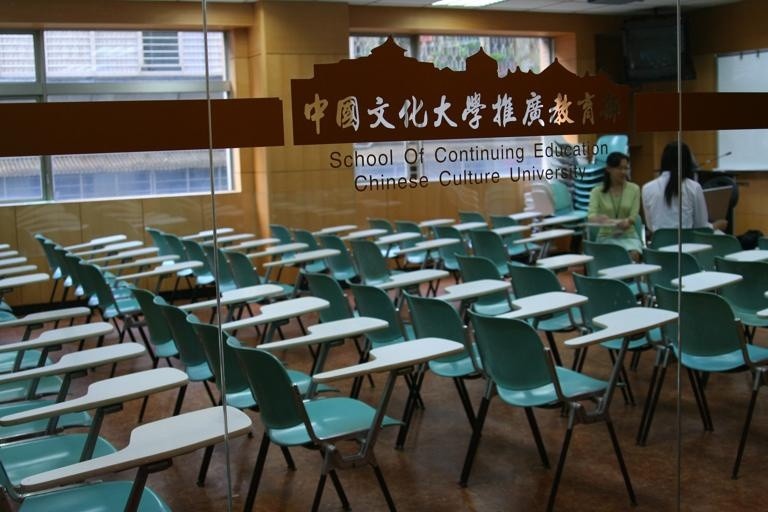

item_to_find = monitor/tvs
[621,15,696,82]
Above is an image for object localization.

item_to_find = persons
[586,150,645,266]
[639,140,730,239]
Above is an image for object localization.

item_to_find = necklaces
[607,184,624,220]
